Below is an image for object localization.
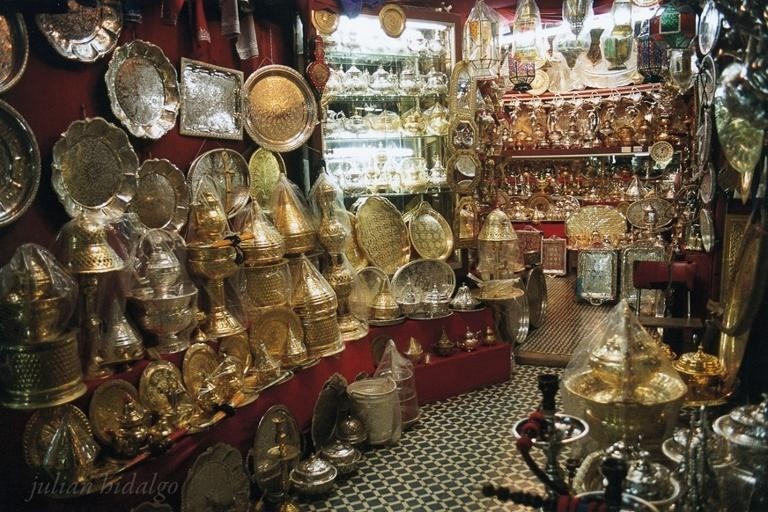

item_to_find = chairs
[632,260,703,350]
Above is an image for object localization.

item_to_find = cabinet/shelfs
[303,2,471,281]
[496,83,663,246]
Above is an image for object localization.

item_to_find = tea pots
[326,64,446,192]
